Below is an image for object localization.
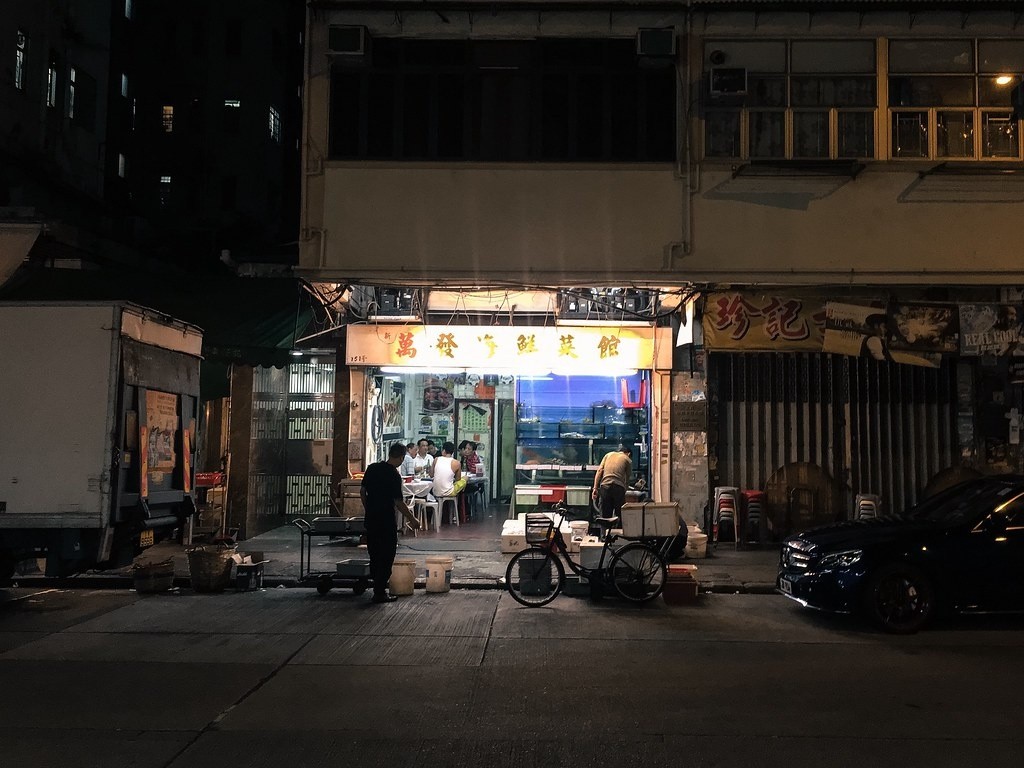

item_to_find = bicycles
[506,499,667,608]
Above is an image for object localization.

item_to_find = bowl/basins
[414,479,421,483]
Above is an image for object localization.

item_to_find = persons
[591,447,634,542]
[459,440,482,521]
[360,443,422,602]
[413,438,434,477]
[397,442,415,477]
[427,440,442,460]
[429,442,469,523]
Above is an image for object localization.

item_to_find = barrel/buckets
[685,533,708,559]
[388,558,417,596]
[424,555,453,592]
[569,520,589,541]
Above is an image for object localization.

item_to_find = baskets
[195,472,222,485]
[132,554,175,593]
[526,514,554,544]
[186,541,236,593]
[197,503,222,529]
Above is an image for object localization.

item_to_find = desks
[350,478,433,537]
[765,461,840,534]
[421,476,489,525]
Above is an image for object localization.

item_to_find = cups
[475,464,484,477]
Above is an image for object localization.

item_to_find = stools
[403,483,485,537]
[621,378,647,408]
[712,486,765,552]
[854,494,880,521]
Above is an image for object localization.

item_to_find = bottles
[462,456,467,471]
[426,459,431,474]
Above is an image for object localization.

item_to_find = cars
[774,474,1024,634]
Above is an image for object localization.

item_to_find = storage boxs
[336,558,371,576]
[349,516,365,531]
[232,551,270,591]
[661,564,699,603]
[541,485,566,502]
[566,485,591,506]
[196,472,222,485]
[207,487,227,504]
[572,536,598,553]
[621,502,679,537]
[313,516,349,531]
[500,512,571,553]
[579,536,643,583]
[515,484,541,505]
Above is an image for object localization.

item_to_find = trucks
[0,299,204,588]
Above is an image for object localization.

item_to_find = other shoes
[452,515,457,524]
[372,592,398,603]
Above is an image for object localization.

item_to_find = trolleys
[291,519,371,595]
